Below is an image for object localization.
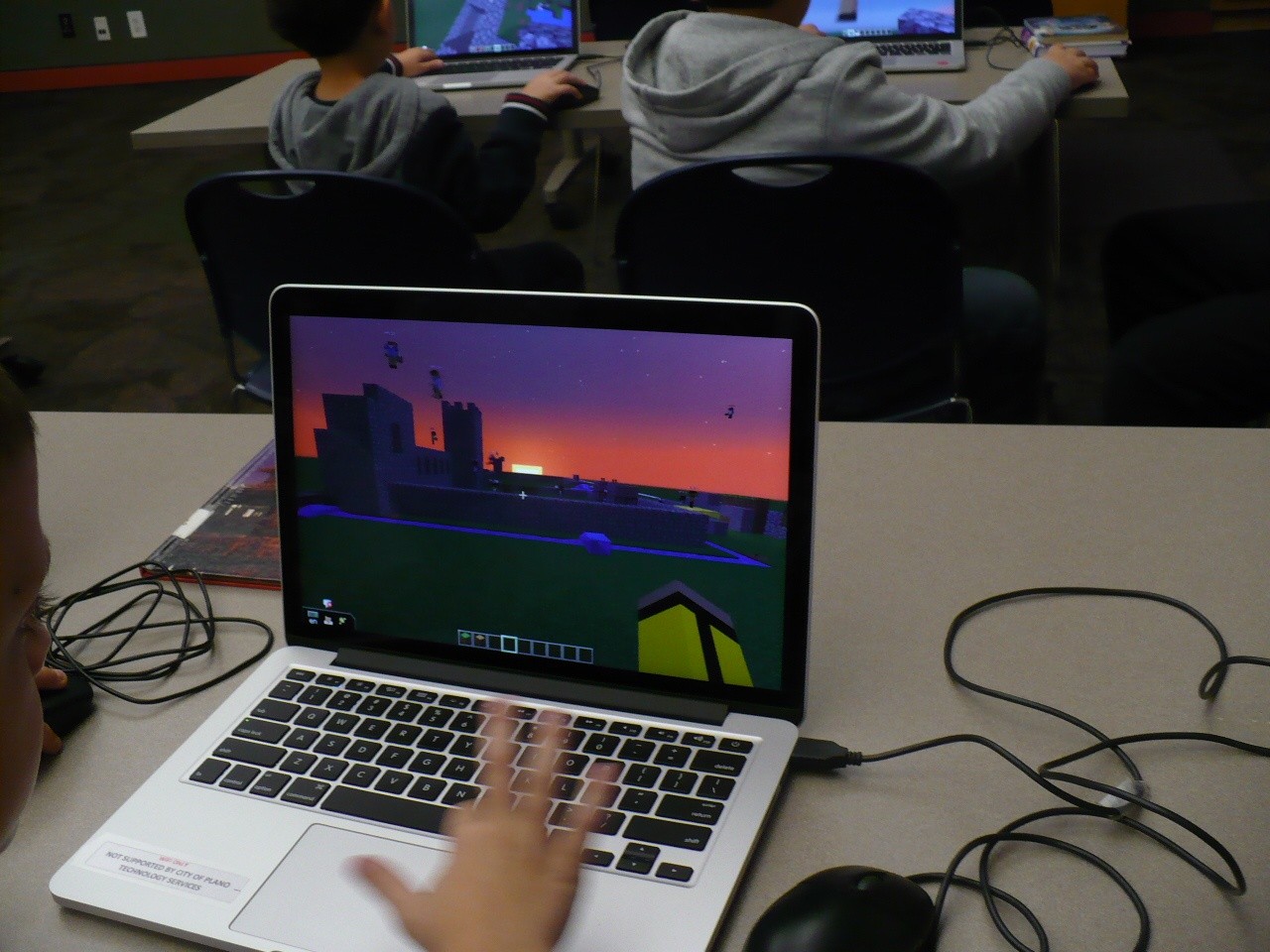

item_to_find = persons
[2,374,623,952]
[265,0,589,292]
[620,1,1100,424]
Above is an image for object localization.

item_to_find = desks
[132,27,1129,287]
[0,410,1270,952]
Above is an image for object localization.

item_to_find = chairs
[1103,197,1269,428]
[185,170,478,413]
[613,150,975,424]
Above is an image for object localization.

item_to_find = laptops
[800,0,966,72]
[49,284,820,952]
[406,0,581,91]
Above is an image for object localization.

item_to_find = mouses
[39,673,94,737]
[551,81,600,109]
[742,866,934,952]
